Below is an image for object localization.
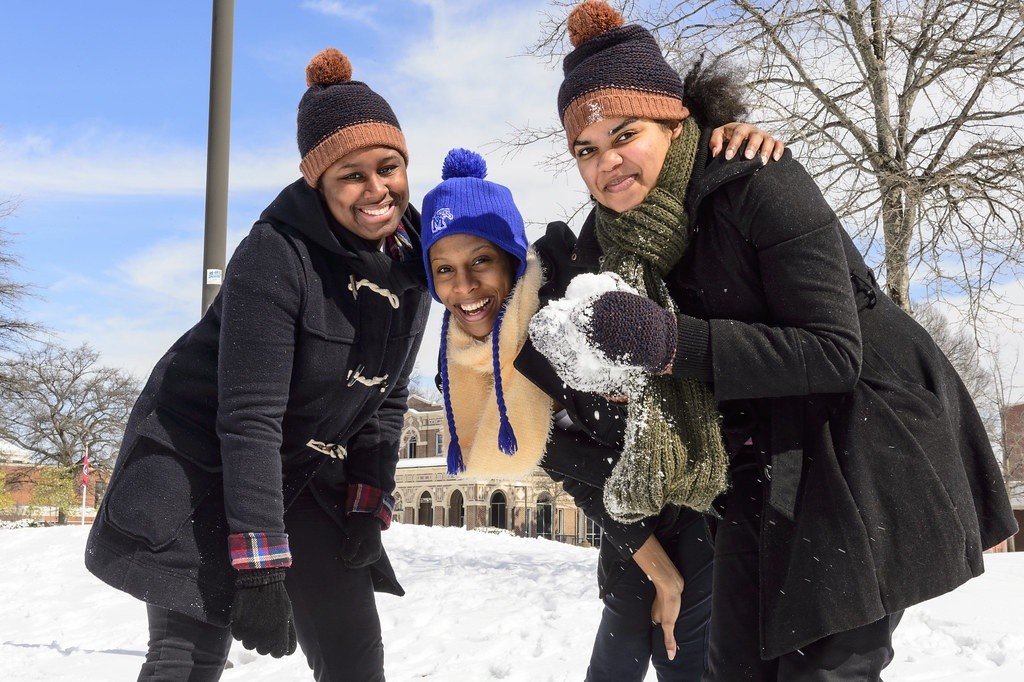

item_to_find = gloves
[342,512,381,568]
[571,291,676,376]
[229,568,297,659]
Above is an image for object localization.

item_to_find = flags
[81,456,89,486]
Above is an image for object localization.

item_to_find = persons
[557,1,1018,682]
[86,51,434,682]
[420,122,788,682]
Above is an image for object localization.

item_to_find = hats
[558,0,690,158]
[420,148,528,477]
[297,48,408,189]
